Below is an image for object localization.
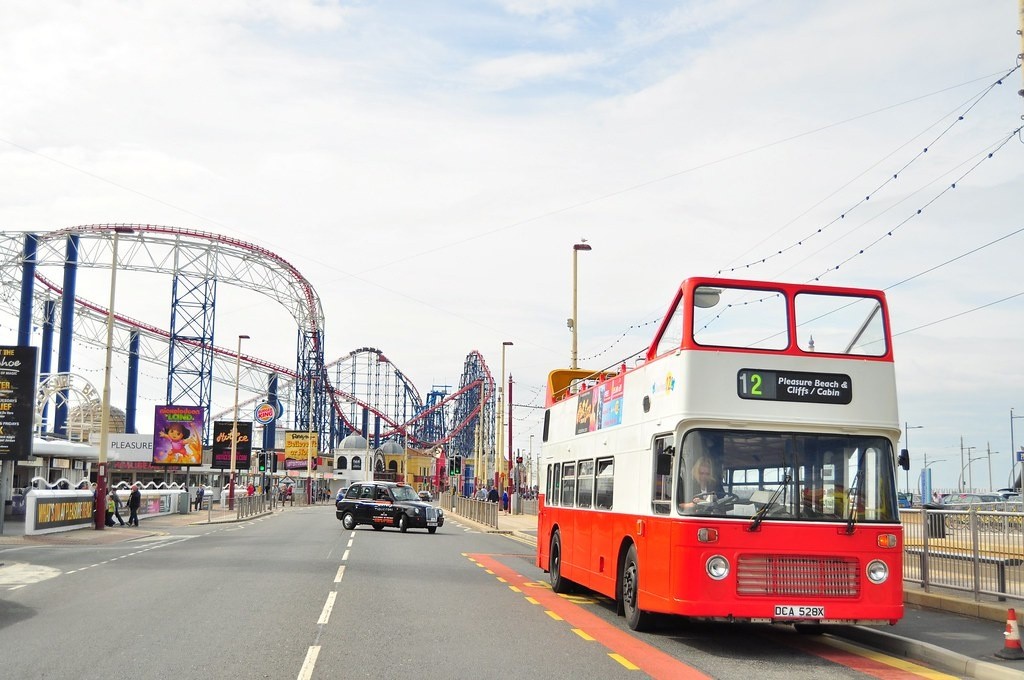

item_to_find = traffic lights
[424,477,427,487]
[556,470,559,488]
[258,453,266,472]
[453,456,463,475]
[449,458,455,477]
[517,457,523,463]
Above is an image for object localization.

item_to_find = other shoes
[132,525,137,527]
[126,523,131,527]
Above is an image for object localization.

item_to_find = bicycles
[944,505,1024,534]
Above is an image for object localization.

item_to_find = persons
[180,482,188,491]
[235,482,262,496]
[475,487,483,499]
[124,484,141,528]
[322,486,327,502]
[502,487,509,516]
[381,490,389,498]
[105,487,125,528]
[932,491,941,503]
[489,487,499,502]
[195,484,204,511]
[313,487,322,502]
[521,485,539,500]
[482,486,486,498]
[272,485,293,507]
[265,483,270,500]
[91,483,97,523]
[327,489,331,502]
[225,485,229,490]
[679,457,725,509]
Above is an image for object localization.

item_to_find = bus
[535,276,910,632]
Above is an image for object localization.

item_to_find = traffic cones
[993,607,1024,660]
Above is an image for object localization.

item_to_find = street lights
[498,342,514,511]
[987,452,1000,492]
[958,456,988,494]
[306,377,321,504]
[918,459,946,494]
[573,244,592,369]
[1011,416,1024,491]
[961,447,976,492]
[530,435,534,490]
[905,426,923,496]
[522,449,525,488]
[95,227,135,531]
[229,335,250,510]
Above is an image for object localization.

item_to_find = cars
[823,488,858,510]
[335,488,359,507]
[417,491,433,502]
[335,480,444,534]
[897,488,1024,511]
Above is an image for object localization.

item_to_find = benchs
[905,549,1023,601]
